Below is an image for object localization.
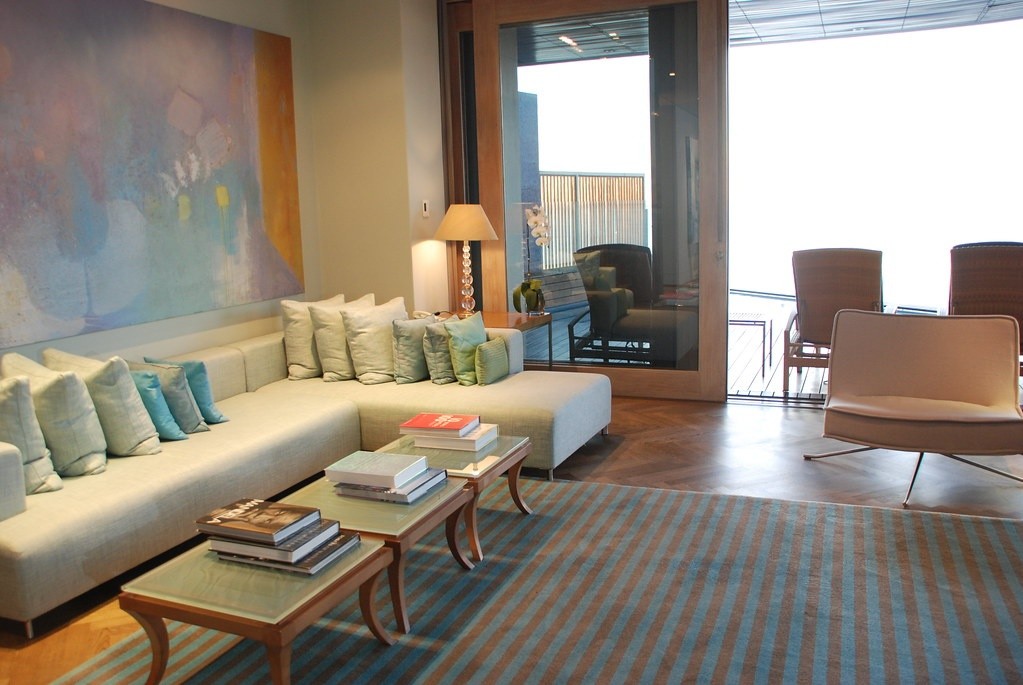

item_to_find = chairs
[783,247,886,397]
[946,239,1023,377]
[567,243,698,367]
[799,307,1023,510]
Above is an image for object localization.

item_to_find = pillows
[423,314,461,385]
[392,312,436,385]
[443,310,486,388]
[143,355,233,423]
[279,292,346,380]
[42,347,163,456]
[0,374,64,493]
[125,358,212,434]
[130,369,190,441]
[473,336,510,388]
[1,351,108,477]
[306,292,376,382]
[340,296,406,385]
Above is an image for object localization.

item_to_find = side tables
[433,309,555,371]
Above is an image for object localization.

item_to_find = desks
[729,311,774,379]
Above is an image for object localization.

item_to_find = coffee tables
[376,426,536,563]
[277,473,480,635]
[119,536,398,685]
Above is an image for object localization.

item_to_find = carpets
[46,474,1023,685]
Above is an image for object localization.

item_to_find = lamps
[432,204,499,320]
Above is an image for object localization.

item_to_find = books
[195,498,362,576]
[399,412,499,452]
[324,450,447,506]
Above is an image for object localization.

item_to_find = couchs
[0,327,615,646]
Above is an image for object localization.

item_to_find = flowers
[509,200,561,314]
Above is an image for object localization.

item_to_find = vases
[527,278,546,316]
